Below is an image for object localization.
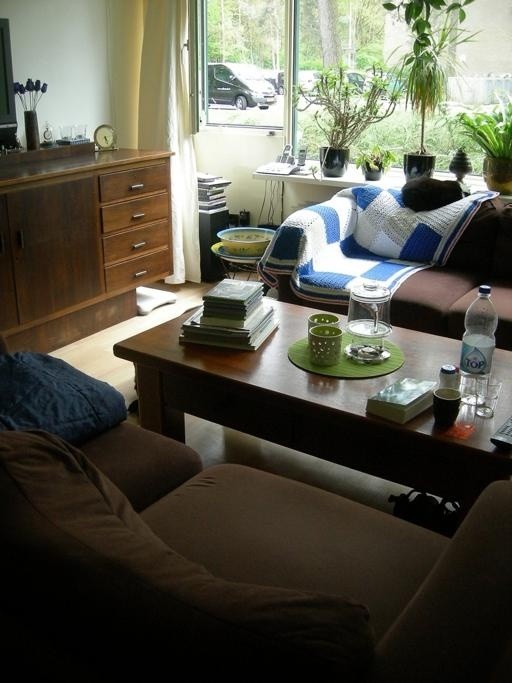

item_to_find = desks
[251,161,486,226]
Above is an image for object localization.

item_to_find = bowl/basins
[216,227,275,256]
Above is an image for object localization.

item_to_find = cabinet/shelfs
[0,162,103,359]
[96,142,181,342]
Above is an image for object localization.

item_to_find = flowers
[13,79,47,111]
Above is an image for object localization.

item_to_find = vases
[24,110,40,151]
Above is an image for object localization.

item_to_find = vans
[208,63,276,111]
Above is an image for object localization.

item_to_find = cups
[433,388,461,427]
[60,126,71,140]
[309,325,343,366]
[308,313,340,344]
[74,124,87,140]
[475,373,503,419]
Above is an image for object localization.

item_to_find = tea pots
[344,281,393,338]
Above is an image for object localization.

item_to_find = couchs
[0,340,512,683]
[258,184,512,352]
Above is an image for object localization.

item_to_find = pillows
[0,429,382,682]
[0,348,127,435]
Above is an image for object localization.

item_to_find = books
[367,377,437,424]
[198,173,232,215]
[179,278,280,353]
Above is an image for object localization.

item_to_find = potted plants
[381,0,484,180]
[291,59,402,177]
[355,146,396,181]
[456,102,512,195]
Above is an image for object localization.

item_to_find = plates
[343,343,390,364]
[211,242,261,264]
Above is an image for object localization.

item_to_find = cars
[265,72,285,95]
[344,73,365,94]
[299,71,324,94]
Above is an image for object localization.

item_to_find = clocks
[94,125,117,154]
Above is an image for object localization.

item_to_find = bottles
[454,366,461,389]
[439,365,456,389]
[459,285,498,406]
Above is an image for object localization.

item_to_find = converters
[240,211,250,225]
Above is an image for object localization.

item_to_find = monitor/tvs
[0,17,18,137]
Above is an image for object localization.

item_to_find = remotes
[490,415,512,449]
